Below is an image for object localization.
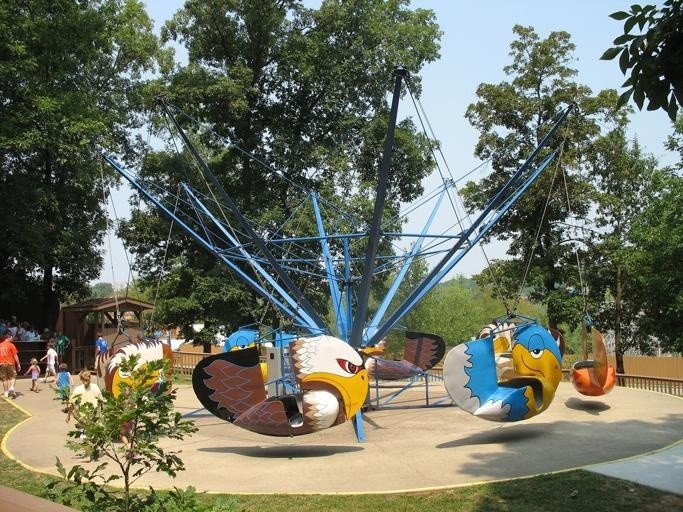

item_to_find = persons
[65,369,102,462]
[119,386,137,450]
[0,315,107,403]
